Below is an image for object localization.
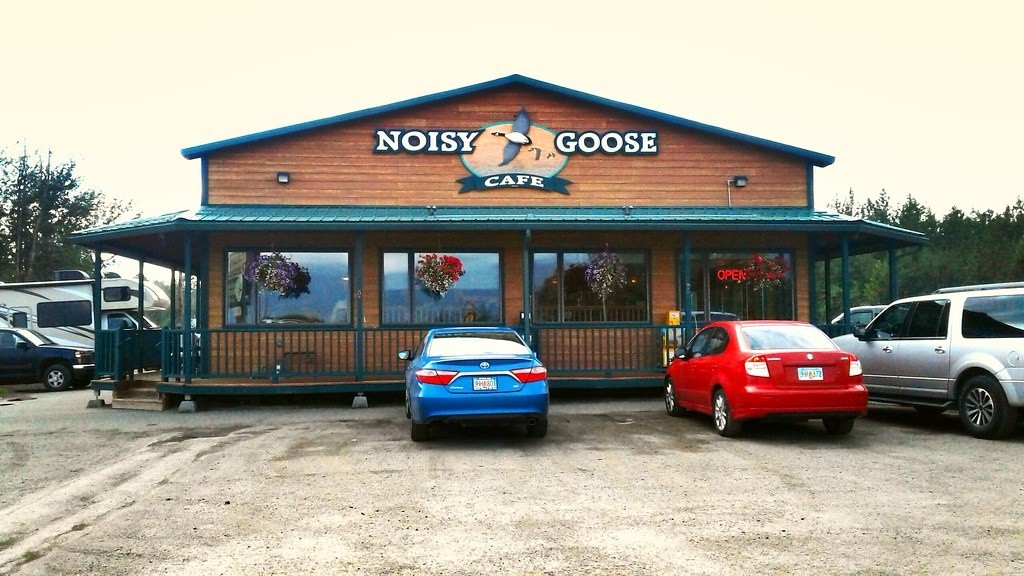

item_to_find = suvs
[831,286,1024,439]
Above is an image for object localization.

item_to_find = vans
[820,304,909,326]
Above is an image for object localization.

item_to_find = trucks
[0,269,171,352]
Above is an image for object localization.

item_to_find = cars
[0,326,94,391]
[396,326,550,442]
[680,310,737,324]
[664,319,868,438]
